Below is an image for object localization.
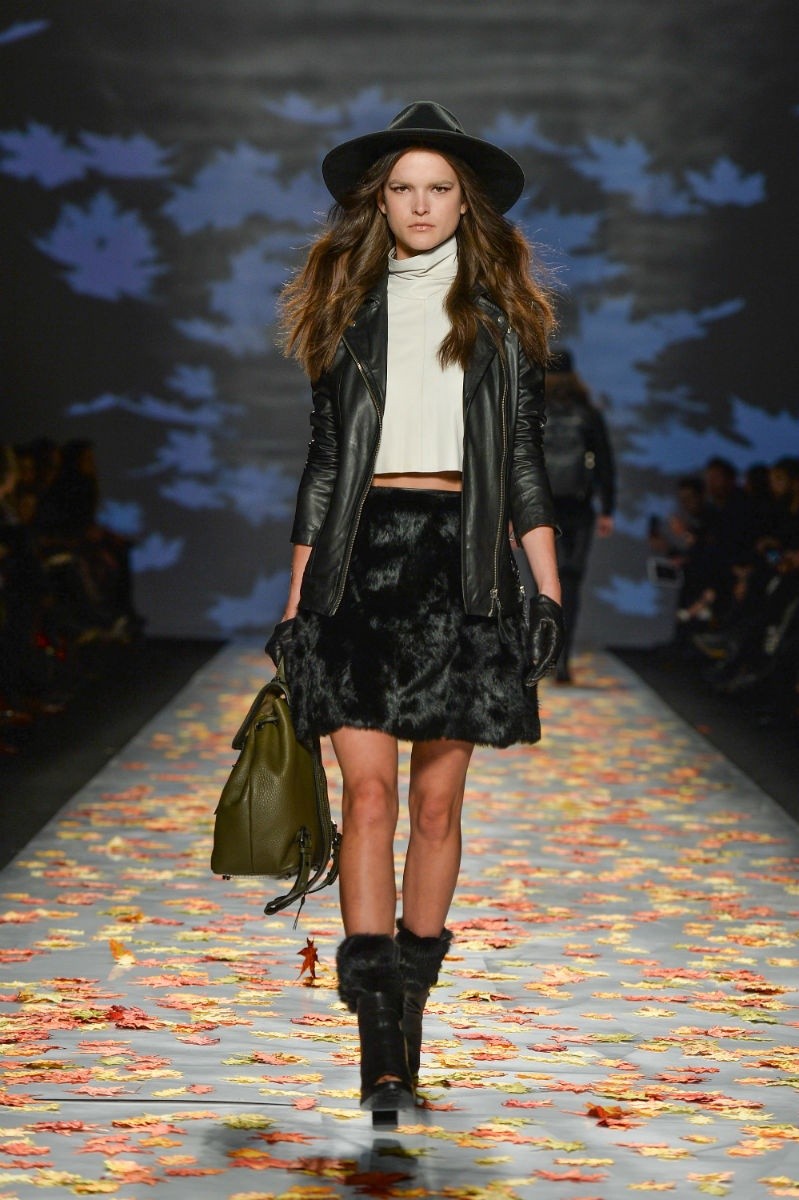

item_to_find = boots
[394,917,453,1081]
[336,934,416,1130]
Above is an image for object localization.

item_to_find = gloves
[525,593,565,687]
[264,616,295,669]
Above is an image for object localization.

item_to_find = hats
[321,101,526,215]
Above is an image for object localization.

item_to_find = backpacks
[210,655,344,914]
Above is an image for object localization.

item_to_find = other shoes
[554,666,574,685]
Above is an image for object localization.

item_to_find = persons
[526,352,618,686]
[264,101,565,1132]
[0,436,146,757]
[647,455,797,730]
